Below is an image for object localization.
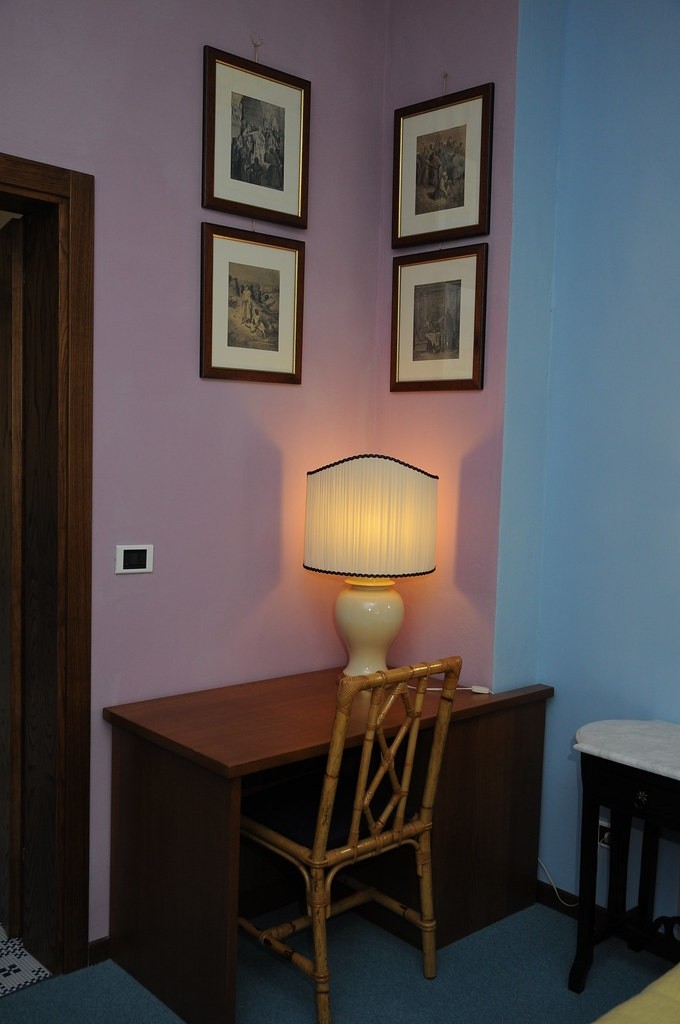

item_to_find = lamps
[301,454,440,690]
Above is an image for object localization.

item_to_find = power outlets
[598,820,611,849]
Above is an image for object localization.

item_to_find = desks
[101,664,555,1024]
[567,718,680,994]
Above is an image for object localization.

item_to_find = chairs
[240,655,462,1024]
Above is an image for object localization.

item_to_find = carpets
[0,921,54,1000]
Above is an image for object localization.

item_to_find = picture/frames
[389,242,489,393]
[391,82,497,251]
[198,220,306,385]
[202,44,311,231]
